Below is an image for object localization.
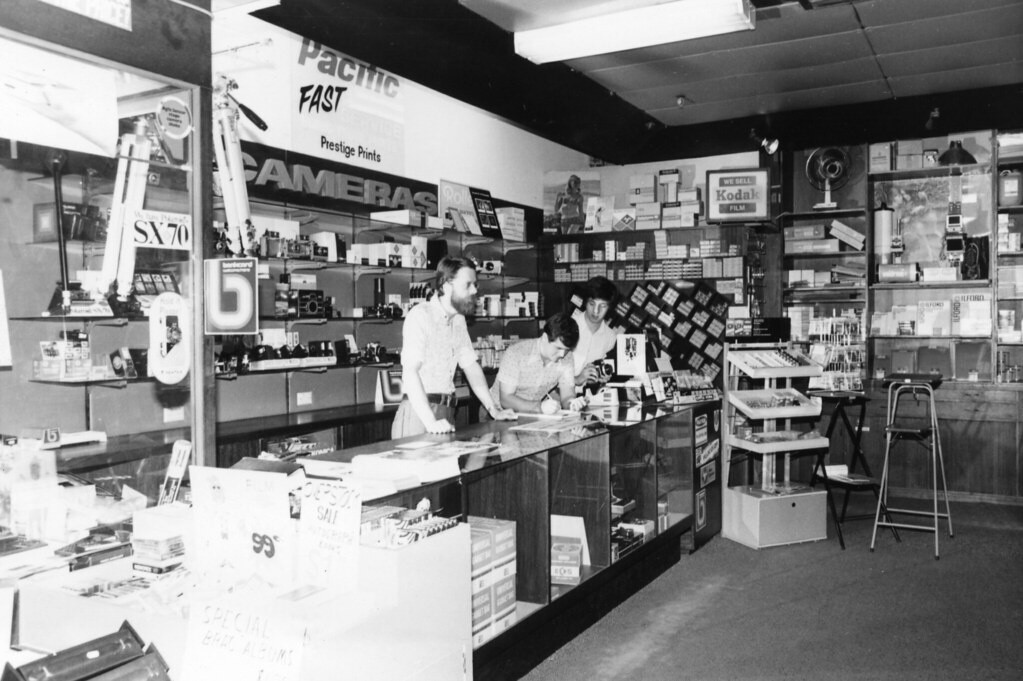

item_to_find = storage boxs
[279,273,317,289]
[274,290,324,318]
[349,242,403,268]
[867,294,991,334]
[33,202,97,240]
[496,207,526,243]
[614,169,702,229]
[550,536,583,586]
[370,211,422,233]
[458,517,517,649]
[555,228,745,281]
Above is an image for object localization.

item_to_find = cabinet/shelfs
[0,396,721,681]
[870,372,953,559]
[780,129,1023,509]
[806,388,900,550]
[10,235,538,385]
[545,226,751,283]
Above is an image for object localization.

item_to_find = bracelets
[487,405,497,413]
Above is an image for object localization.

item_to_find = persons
[478,310,590,425]
[554,175,584,235]
[565,274,622,401]
[390,251,519,438]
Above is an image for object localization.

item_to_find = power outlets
[163,406,184,423]
[297,392,312,407]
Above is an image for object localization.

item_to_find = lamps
[745,126,779,155]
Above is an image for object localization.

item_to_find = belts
[402,392,459,408]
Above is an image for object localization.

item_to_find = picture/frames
[706,168,771,223]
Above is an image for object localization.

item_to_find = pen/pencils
[546,392,553,399]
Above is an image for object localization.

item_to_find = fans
[804,145,851,210]
